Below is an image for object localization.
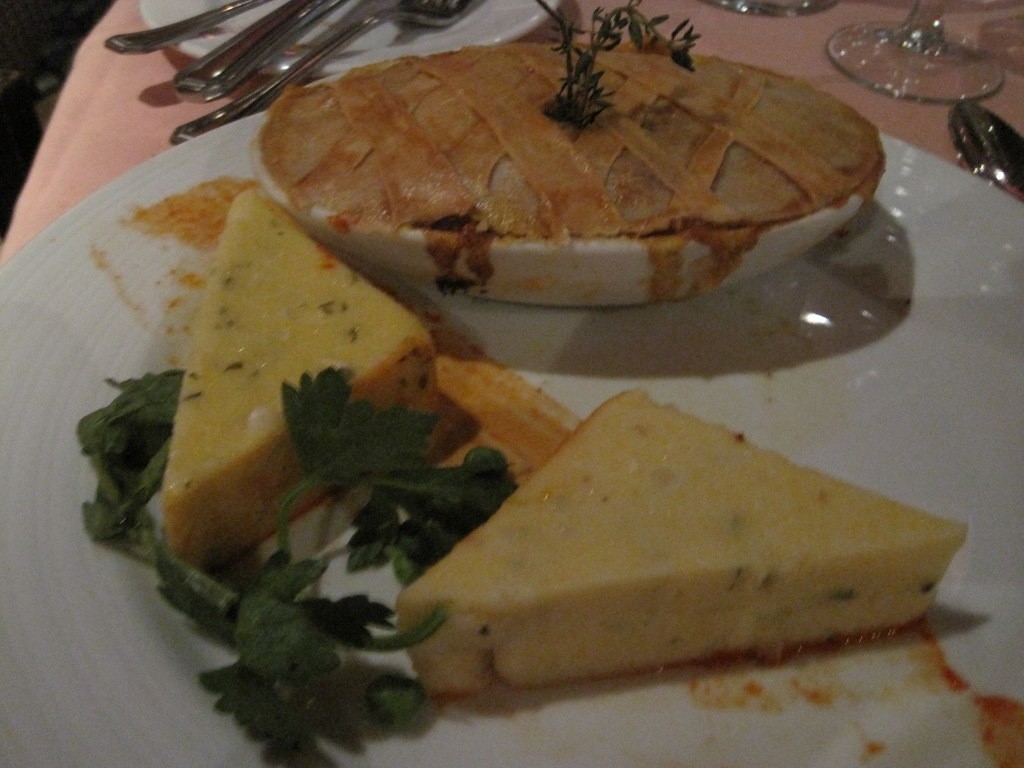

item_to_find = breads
[158,190,437,566]
[254,41,878,240]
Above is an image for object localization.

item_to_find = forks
[171,0,474,140]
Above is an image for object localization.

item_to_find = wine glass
[826,0,1004,104]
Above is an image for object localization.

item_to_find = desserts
[393,389,968,697]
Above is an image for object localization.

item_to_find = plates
[136,0,561,74]
[0,107,1024,768]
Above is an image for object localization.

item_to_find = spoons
[950,102,1024,197]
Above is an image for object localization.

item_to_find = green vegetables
[539,0,705,126]
[73,362,518,768]
